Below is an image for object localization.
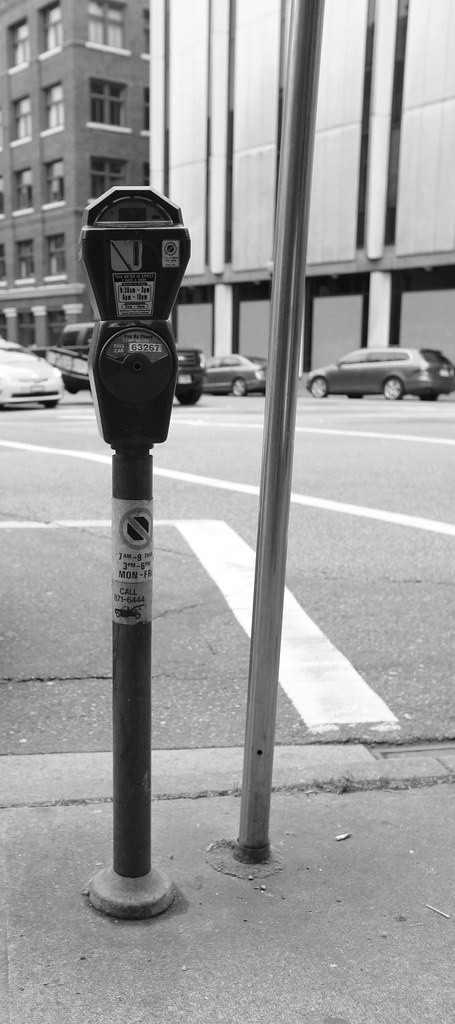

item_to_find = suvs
[53,319,208,405]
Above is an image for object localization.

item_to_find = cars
[0,339,65,412]
[305,346,455,401]
[201,352,267,398]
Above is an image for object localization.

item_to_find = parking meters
[77,183,195,876]
